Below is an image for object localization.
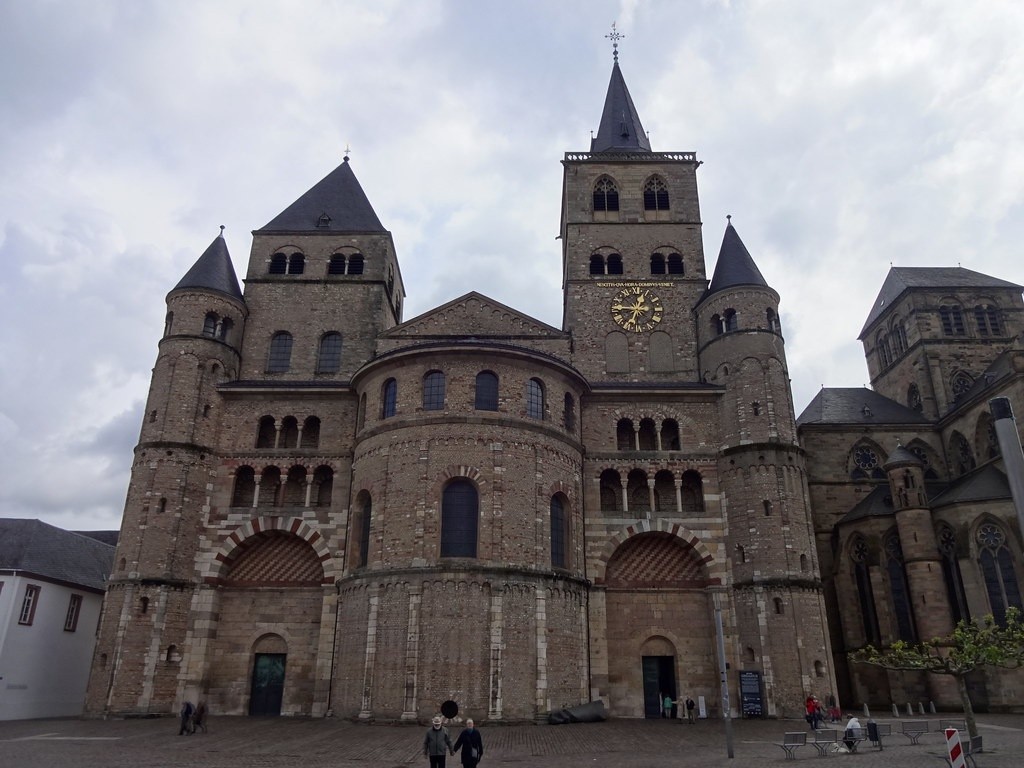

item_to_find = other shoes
[847,745,857,754]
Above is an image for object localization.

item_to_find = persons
[664,695,672,719]
[670,696,685,723]
[686,696,695,724]
[179,700,208,736]
[659,692,663,720]
[423,717,453,768]
[805,694,843,730]
[452,719,483,768]
[842,714,862,754]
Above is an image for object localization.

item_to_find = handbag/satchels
[683,714,685,717]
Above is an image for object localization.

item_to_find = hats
[847,714,853,718]
[433,717,442,725]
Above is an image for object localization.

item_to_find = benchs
[934,718,967,736]
[837,727,868,754]
[897,721,928,744]
[937,735,983,768]
[773,732,807,761]
[865,724,892,747]
[806,729,837,757]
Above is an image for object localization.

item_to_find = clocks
[611,286,664,333]
